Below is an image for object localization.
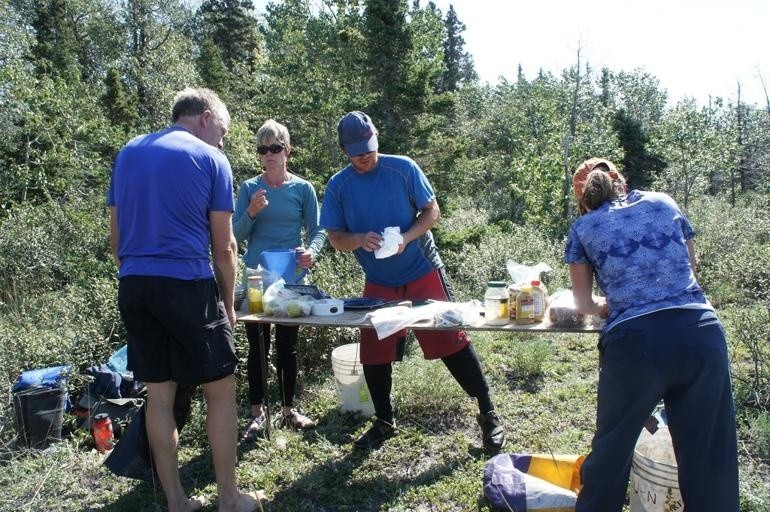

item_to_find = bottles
[92,412,114,451]
[484,280,546,326]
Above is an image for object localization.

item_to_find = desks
[237,300,606,440]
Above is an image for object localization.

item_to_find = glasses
[258,144,284,154]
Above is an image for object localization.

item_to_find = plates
[341,298,386,309]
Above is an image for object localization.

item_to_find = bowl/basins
[234,287,245,311]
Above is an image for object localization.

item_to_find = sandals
[243,413,266,438]
[283,410,316,429]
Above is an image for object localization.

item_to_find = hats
[574,157,619,199]
[338,110,379,157]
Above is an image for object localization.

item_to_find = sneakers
[476,409,506,448]
[353,417,396,448]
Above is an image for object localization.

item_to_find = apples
[287,302,299,317]
[265,308,273,316]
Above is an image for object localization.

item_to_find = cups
[258,248,312,288]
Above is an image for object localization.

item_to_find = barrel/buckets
[331,328,374,416]
[631,429,685,510]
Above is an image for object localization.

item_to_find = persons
[232,119,320,435]
[106,87,271,511]
[319,111,508,450]
[563,157,739,512]
